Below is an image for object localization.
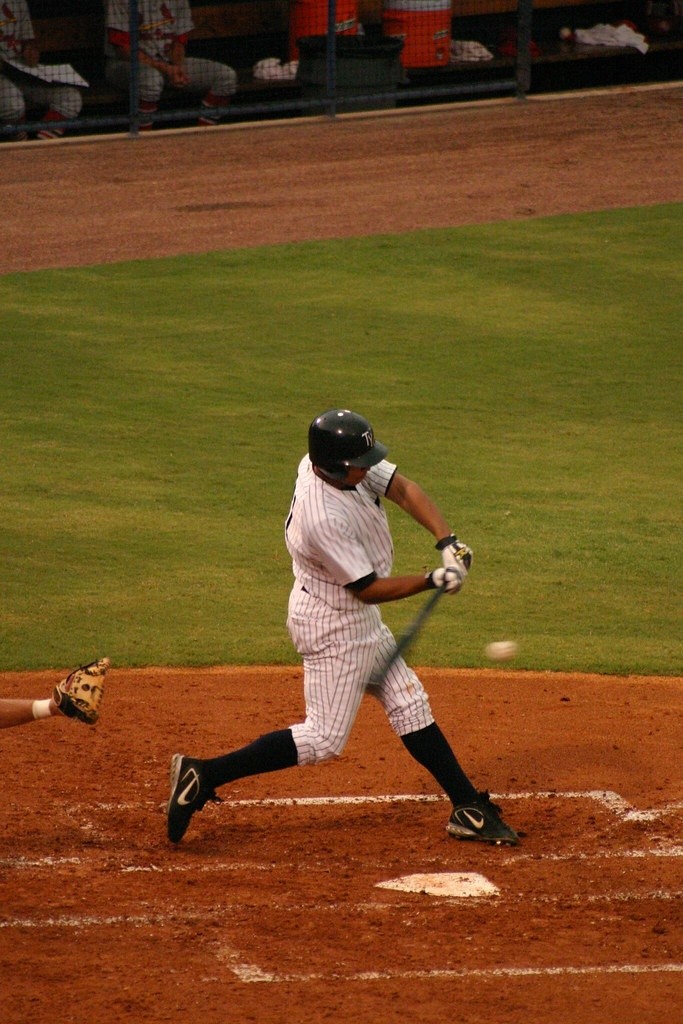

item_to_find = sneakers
[446,790,518,846]
[166,753,226,843]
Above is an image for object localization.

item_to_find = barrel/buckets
[294,35,403,115]
[379,0,452,68]
[287,0,359,61]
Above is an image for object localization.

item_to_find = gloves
[435,533,472,577]
[424,568,461,595]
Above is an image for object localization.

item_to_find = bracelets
[32,700,52,719]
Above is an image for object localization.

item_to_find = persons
[103,0,238,131]
[0,0,83,143]
[0,655,111,732]
[167,409,525,850]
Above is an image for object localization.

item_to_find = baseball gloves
[52,655,112,725]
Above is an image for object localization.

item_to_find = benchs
[0,0,683,113]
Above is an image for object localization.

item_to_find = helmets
[308,409,387,480]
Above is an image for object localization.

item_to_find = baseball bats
[365,577,448,693]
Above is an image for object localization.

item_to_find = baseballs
[486,640,515,662]
